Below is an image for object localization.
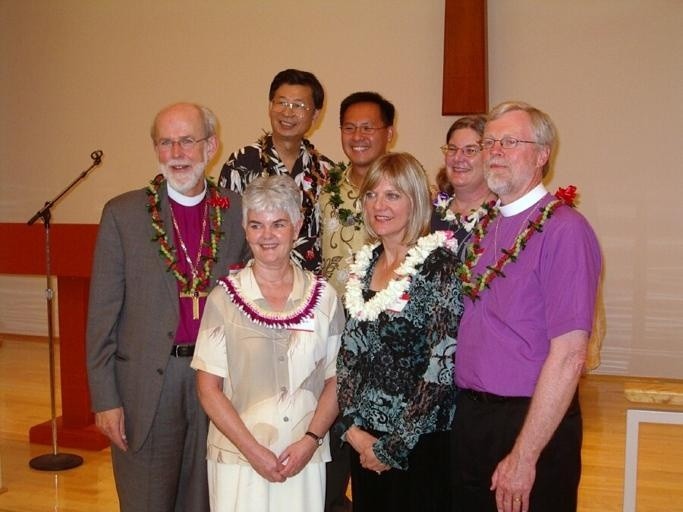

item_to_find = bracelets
[303,432,324,447]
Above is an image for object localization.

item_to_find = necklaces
[164,199,212,320]
[492,201,544,265]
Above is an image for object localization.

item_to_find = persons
[331,149,467,512]
[448,101,604,512]
[215,69,340,275]
[83,99,253,511]
[187,174,346,511]
[427,112,498,247]
[315,89,396,511]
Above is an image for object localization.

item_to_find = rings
[512,497,521,503]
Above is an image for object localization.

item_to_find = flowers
[555,185,580,209]
[344,230,459,323]
[432,191,495,233]
[204,196,230,209]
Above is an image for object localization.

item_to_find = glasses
[155,135,207,152]
[478,136,537,150]
[440,143,482,158]
[339,122,386,136]
[270,98,316,114]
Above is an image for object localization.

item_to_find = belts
[171,343,196,359]
